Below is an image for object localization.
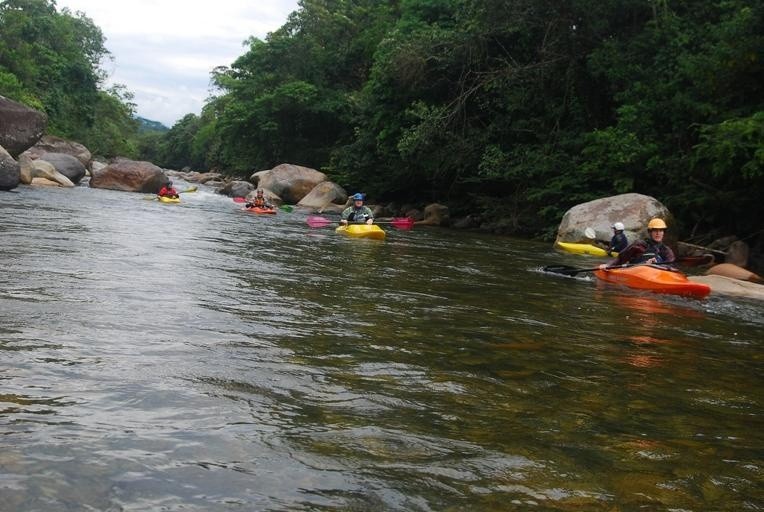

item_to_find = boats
[335,224,385,240]
[557,241,619,258]
[247,207,277,215]
[158,195,182,203]
[593,263,711,301]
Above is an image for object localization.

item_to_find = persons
[246,189,273,209]
[599,218,675,270]
[339,193,374,225]
[159,183,177,196]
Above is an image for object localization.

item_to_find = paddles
[584,228,607,249]
[543,256,713,277]
[307,216,415,230]
[144,186,197,200]
[234,197,270,207]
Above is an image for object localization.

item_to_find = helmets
[354,193,364,200]
[648,218,668,229]
[257,189,263,193]
[612,222,624,230]
